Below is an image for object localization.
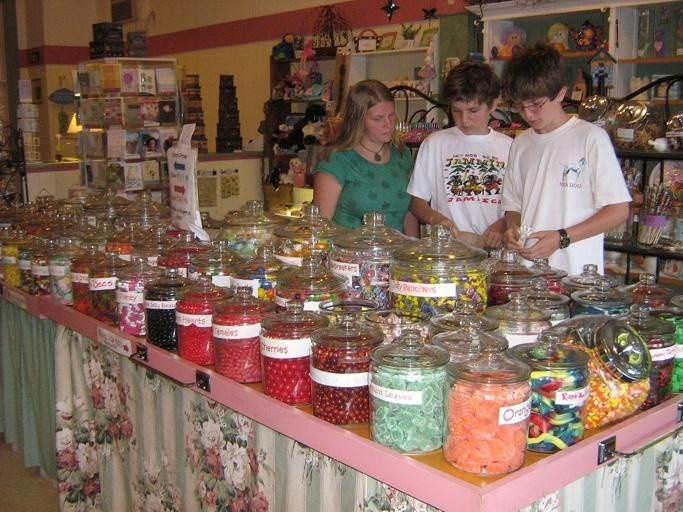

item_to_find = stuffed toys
[574,23,600,52]
[265,32,335,193]
[490,28,526,59]
[417,55,437,97]
[543,23,571,54]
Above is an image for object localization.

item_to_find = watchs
[557,228,569,250]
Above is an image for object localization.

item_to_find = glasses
[511,99,549,113]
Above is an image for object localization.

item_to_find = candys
[0,168,683,473]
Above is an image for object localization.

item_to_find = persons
[497,42,635,279]
[406,59,515,260]
[309,80,420,241]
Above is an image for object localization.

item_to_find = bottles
[578,94,683,152]
[0,187,682,476]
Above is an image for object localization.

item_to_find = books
[71,62,179,208]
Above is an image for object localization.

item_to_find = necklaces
[359,142,384,162]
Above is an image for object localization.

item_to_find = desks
[0,278,683,512]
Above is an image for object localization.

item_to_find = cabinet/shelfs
[77,54,182,197]
[261,41,346,186]
[387,70,683,279]
[462,0,682,120]
[346,45,443,129]
